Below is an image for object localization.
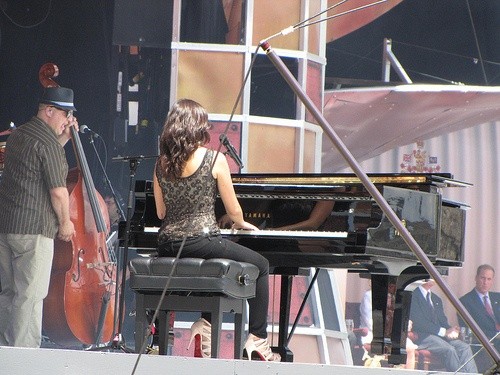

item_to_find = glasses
[46,105,71,118]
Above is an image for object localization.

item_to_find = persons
[456,264,500,358]
[0,88,76,351]
[152,98,283,363]
[407,279,478,374]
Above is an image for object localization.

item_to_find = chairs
[345,302,419,370]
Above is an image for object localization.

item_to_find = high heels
[186,317,211,359]
[246,333,282,362]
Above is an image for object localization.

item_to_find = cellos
[38,62,126,349]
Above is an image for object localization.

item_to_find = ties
[482,296,500,331]
[426,293,432,307]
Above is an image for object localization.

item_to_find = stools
[128,256,260,359]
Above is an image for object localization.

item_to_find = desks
[457,335,494,373]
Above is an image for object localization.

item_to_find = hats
[37,87,77,111]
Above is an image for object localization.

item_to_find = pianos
[118,172,472,363]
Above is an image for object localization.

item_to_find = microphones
[219,134,244,169]
[80,125,102,139]
